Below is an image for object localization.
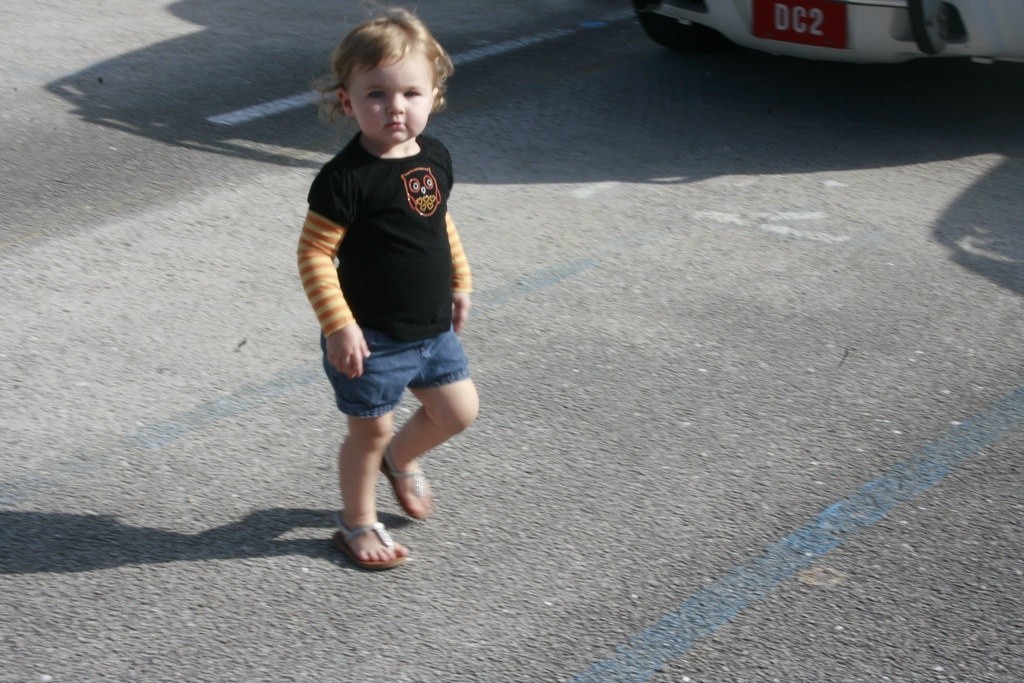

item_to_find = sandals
[333,512,411,568]
[379,448,432,520]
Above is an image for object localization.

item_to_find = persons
[298,9,478,566]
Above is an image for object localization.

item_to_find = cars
[633,0,1022,67]
